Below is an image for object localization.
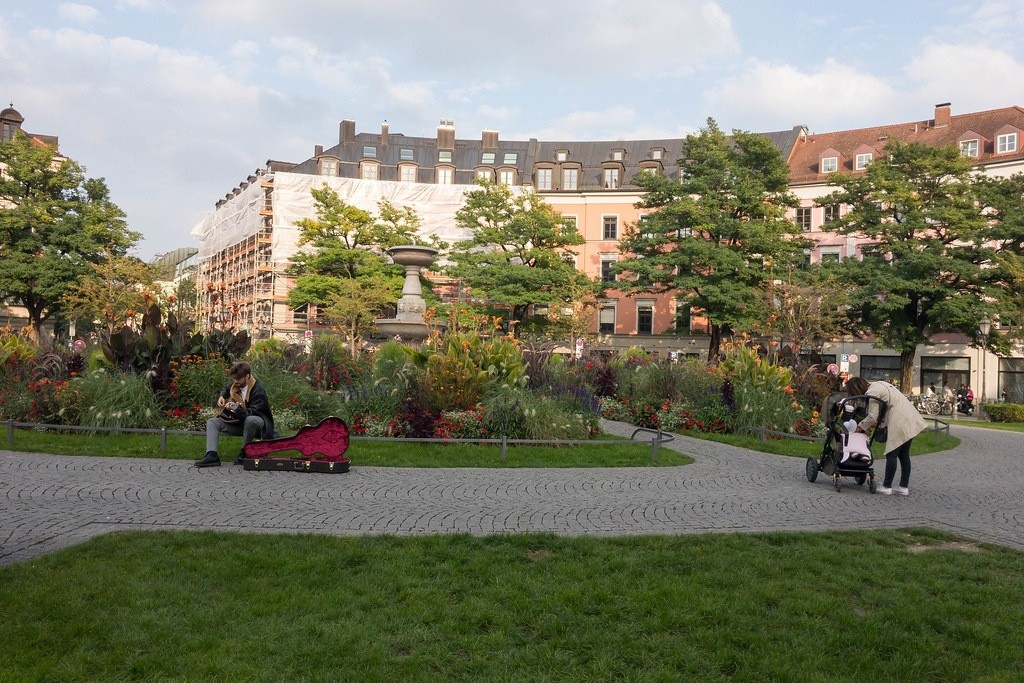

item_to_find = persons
[929,380,1008,412]
[194,362,274,466]
[840,377,928,495]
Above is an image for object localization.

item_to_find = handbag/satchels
[875,426,887,443]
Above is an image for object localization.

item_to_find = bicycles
[908,392,974,416]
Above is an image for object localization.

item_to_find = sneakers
[876,482,909,495]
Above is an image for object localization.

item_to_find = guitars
[216,400,240,418]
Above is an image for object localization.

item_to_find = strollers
[805,393,889,495]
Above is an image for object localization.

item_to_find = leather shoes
[234,452,247,465]
[194,454,221,466]
